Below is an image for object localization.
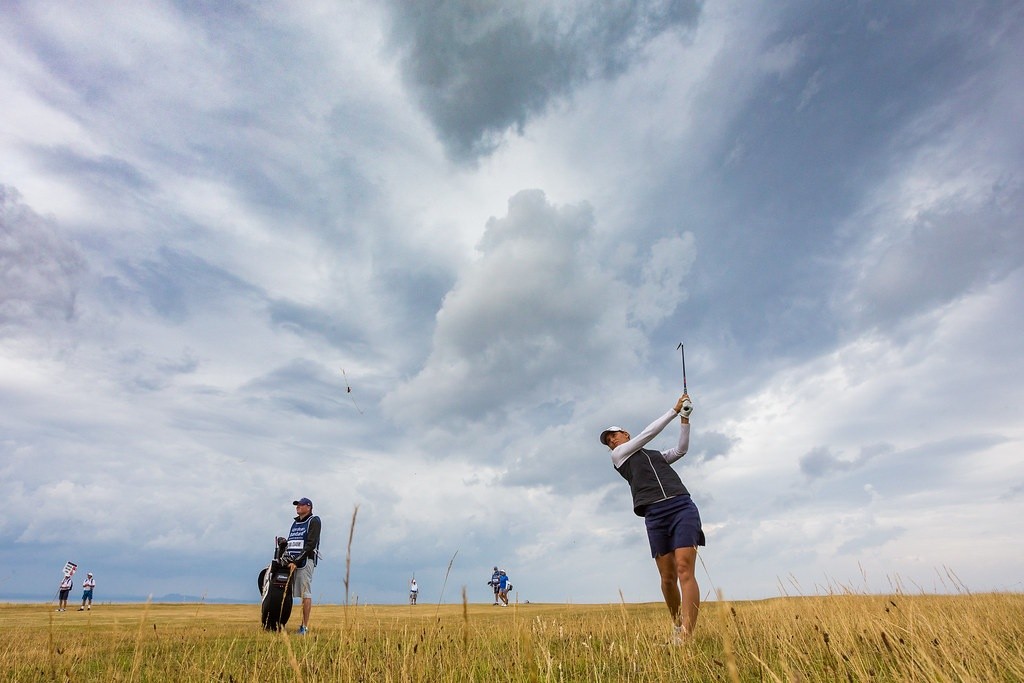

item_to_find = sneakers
[297,626,308,635]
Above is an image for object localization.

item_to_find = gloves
[679,398,694,419]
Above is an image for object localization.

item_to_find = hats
[500,569,506,573]
[293,498,312,507]
[600,426,630,445]
[413,580,416,582]
[88,573,92,576]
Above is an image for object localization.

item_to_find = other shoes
[506,599,508,605]
[77,608,83,611]
[55,609,60,612]
[493,603,498,606]
[501,603,507,607]
[88,608,90,611]
[61,609,66,611]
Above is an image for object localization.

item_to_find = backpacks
[63,579,73,590]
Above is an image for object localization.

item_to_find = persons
[410,579,418,605]
[498,569,508,607]
[56,575,73,611]
[284,498,320,634]
[599,394,706,642]
[77,573,95,611]
[490,567,501,605]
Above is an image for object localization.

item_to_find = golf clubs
[676,341,689,411]
